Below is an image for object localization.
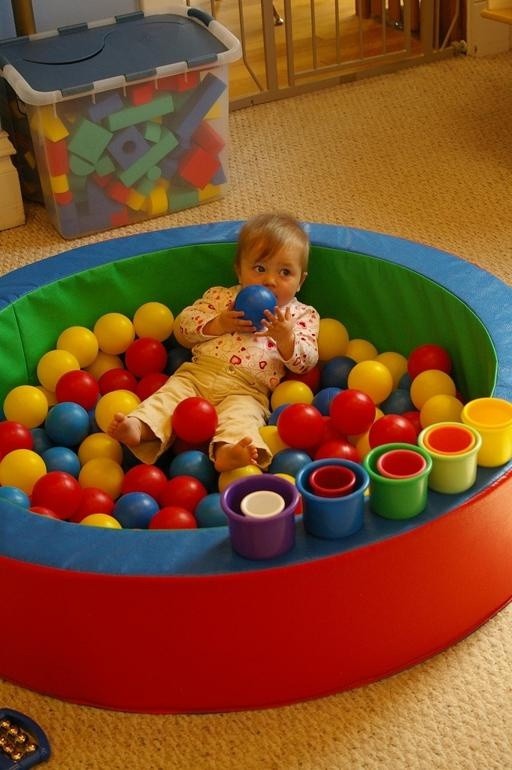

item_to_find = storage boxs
[1,3,242,239]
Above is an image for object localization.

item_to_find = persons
[108,211,319,475]
